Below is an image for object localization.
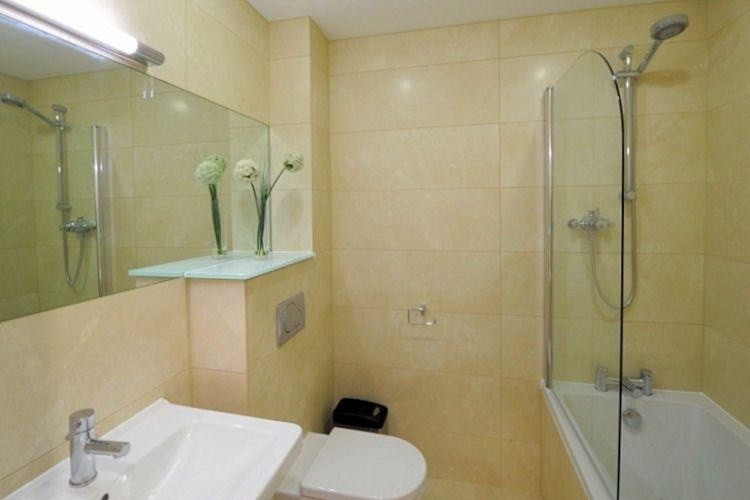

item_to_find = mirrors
[0,13,272,323]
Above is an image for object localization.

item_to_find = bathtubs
[538,378,750,500]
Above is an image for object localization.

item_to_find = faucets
[621,376,643,399]
[65,408,131,488]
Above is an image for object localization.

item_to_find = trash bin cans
[332,398,388,435]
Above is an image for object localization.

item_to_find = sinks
[0,396,304,500]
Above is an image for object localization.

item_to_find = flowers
[192,153,232,263]
[232,149,306,264]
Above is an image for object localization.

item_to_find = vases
[252,197,270,261]
[208,200,228,258]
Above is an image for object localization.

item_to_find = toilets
[274,428,429,500]
